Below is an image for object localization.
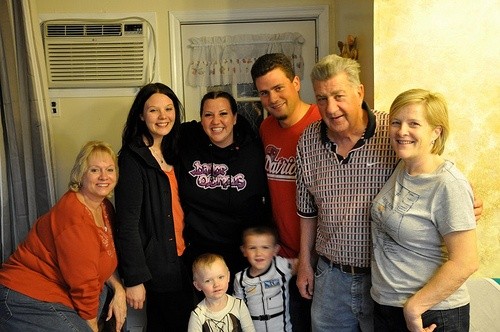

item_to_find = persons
[115,83,268,332]
[251,53,324,332]
[297,54,483,332]
[231,223,299,332]
[370,88,479,332]
[0,142,128,332]
[173,90,269,308]
[187,254,255,331]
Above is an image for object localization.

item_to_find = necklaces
[151,147,164,164]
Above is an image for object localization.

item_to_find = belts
[321,255,371,274]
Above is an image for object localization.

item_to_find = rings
[124,317,127,320]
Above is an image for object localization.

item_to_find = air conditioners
[42,16,158,88]
